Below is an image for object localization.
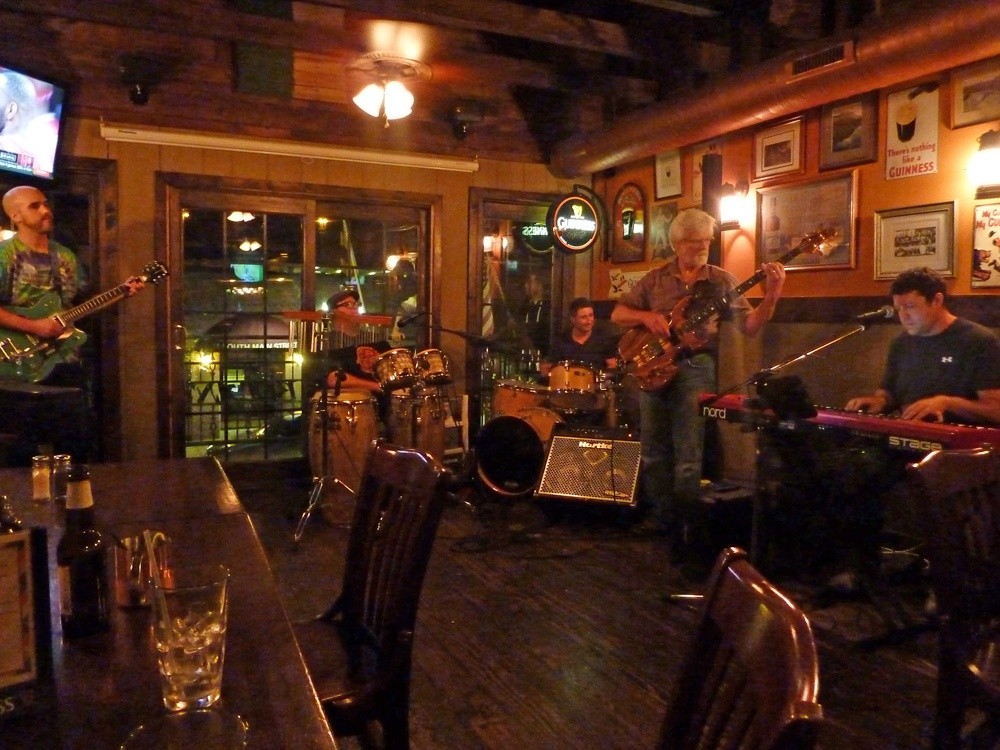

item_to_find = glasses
[682,236,718,249]
[333,300,362,309]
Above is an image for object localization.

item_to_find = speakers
[676,480,754,582]
[534,423,643,522]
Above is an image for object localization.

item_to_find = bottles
[55,466,117,657]
[0,492,37,693]
[26,453,77,639]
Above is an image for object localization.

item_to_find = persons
[0,184,147,465]
[313,288,398,396]
[536,296,624,425]
[609,206,788,538]
[235,366,288,420]
[382,260,552,351]
[820,266,1000,598]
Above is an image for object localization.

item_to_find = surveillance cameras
[455,121,467,139]
[129,84,147,105]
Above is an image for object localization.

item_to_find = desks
[0,454,337,749]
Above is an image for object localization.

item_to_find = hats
[327,291,358,309]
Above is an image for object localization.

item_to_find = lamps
[346,49,433,129]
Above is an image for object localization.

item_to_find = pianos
[701,391,1000,460]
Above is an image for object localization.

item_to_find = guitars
[0,258,170,384]
[619,222,838,393]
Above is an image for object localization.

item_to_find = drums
[549,360,601,415]
[307,388,378,528]
[371,347,416,388]
[472,407,565,498]
[391,387,446,465]
[412,348,452,384]
[494,378,549,416]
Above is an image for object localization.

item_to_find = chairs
[650,546,825,750]
[290,436,453,750]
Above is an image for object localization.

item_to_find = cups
[112,527,173,607]
[146,564,231,711]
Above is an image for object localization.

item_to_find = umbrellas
[194,311,301,369]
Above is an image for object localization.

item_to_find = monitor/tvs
[0,59,70,192]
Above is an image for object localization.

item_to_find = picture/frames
[818,88,879,173]
[873,200,958,281]
[652,145,685,202]
[750,112,806,184]
[968,201,1000,289]
[949,53,1000,130]
[754,169,858,275]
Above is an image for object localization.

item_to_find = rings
[770,270,776,274]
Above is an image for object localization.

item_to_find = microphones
[397,311,426,328]
[857,305,894,322]
